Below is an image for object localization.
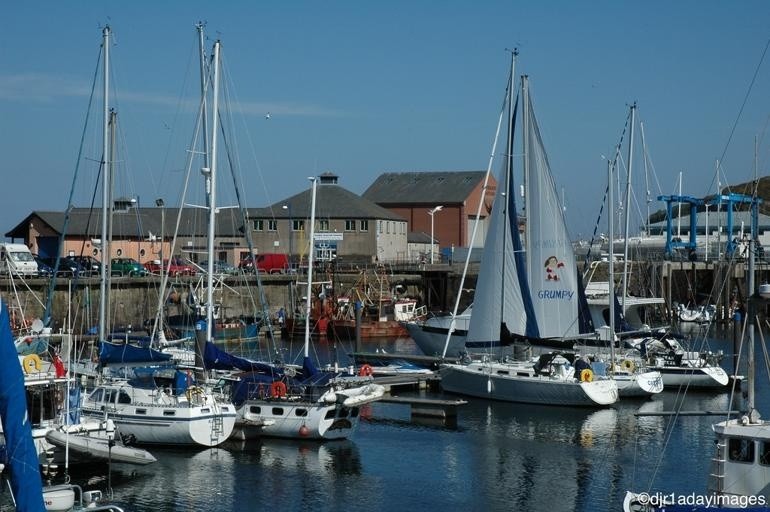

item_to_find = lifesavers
[271,382,285,397]
[620,360,634,372]
[361,365,373,376]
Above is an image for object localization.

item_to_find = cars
[0,243,295,277]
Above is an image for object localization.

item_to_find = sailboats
[0,16,770,512]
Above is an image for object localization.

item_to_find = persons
[275,306,286,328]
